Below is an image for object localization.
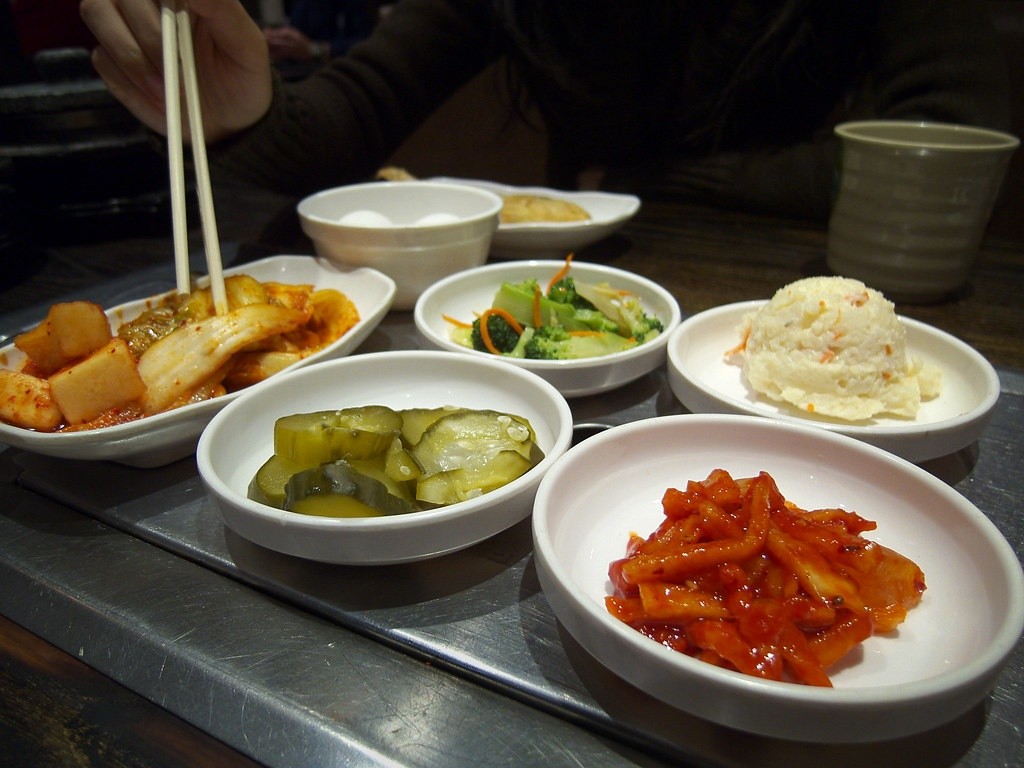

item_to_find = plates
[0,255,397,471]
[430,176,641,252]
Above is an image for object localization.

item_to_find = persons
[80,0,1024,226]
[263,25,359,71]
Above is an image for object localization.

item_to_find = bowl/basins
[415,258,682,401]
[532,412,1024,743]
[667,299,1001,464]
[196,350,572,567]
[297,181,504,312]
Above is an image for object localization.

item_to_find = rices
[724,276,940,420]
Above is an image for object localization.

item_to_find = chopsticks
[162,8,229,318]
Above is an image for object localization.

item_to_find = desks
[0,189,1024,768]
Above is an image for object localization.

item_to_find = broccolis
[449,277,663,361]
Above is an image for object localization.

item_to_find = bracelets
[310,39,321,63]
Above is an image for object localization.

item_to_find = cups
[823,120,1021,303]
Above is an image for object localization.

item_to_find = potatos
[0,301,147,430]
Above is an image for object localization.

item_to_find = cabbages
[118,272,361,414]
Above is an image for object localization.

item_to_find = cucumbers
[249,405,545,517]
